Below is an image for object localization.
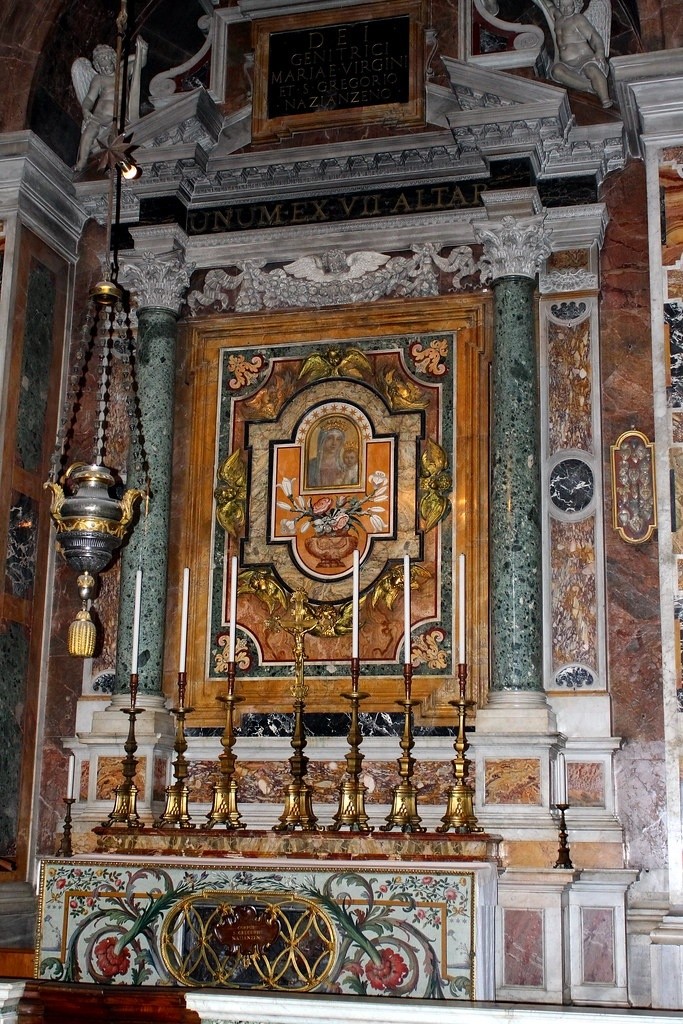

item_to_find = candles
[458,553,466,664]
[131,566,142,674]
[404,553,411,663]
[352,549,359,658]
[559,753,566,804]
[67,754,75,799]
[229,555,238,662]
[179,566,189,672]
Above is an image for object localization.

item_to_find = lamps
[42,0,150,657]
[9,496,37,544]
